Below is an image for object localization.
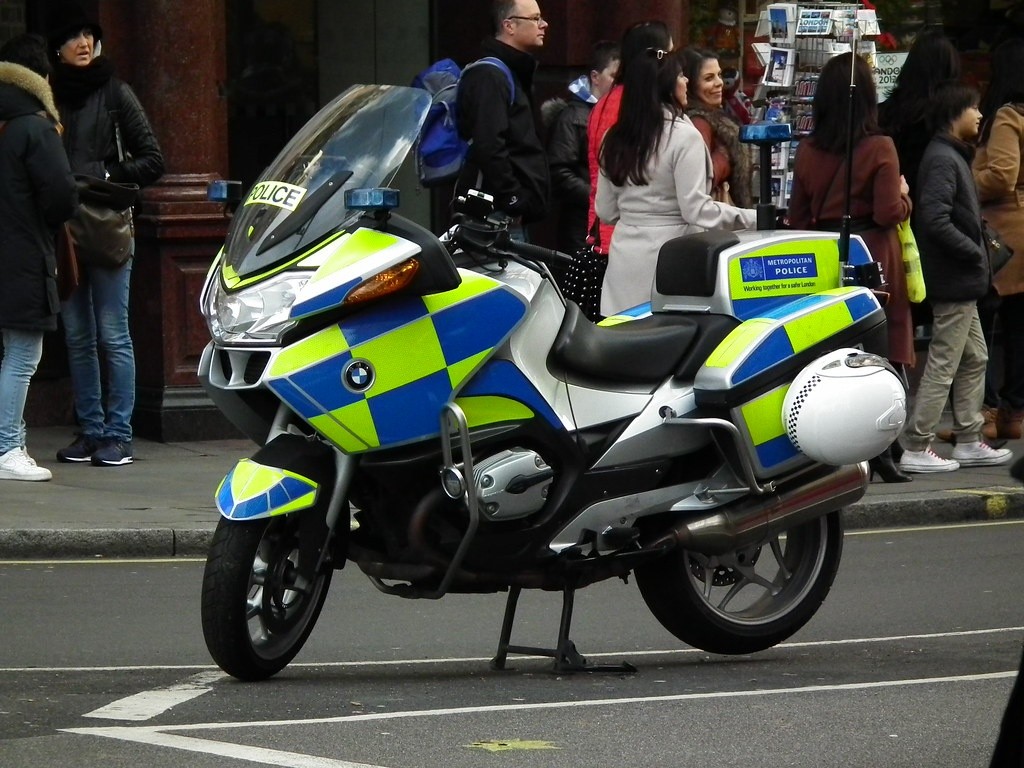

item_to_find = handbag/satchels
[57,220,78,298]
[897,217,926,302]
[70,175,139,268]
[562,217,608,322]
[984,225,1014,272]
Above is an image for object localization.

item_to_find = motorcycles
[196,82,896,685]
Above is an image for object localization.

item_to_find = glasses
[506,15,543,25]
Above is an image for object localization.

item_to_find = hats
[2,34,50,77]
[47,10,103,62]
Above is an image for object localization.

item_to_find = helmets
[782,347,907,466]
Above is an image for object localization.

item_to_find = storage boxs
[693,284,891,480]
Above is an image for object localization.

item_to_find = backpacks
[409,57,517,187]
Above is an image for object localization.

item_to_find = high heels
[889,439,904,462]
[868,444,914,482]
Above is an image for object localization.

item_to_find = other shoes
[999,401,1024,438]
[936,406,998,441]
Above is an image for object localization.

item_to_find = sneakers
[0,447,53,483]
[58,432,101,462]
[92,436,133,467]
[898,445,961,473]
[951,440,1012,466]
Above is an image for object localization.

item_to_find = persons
[789,53,916,483]
[678,45,751,207]
[550,40,621,215]
[41,3,165,465]
[451,0,551,236]
[594,49,757,317]
[876,30,962,325]
[586,19,674,255]
[0,35,90,481]
[936,35,1024,439]
[898,79,1013,473]
